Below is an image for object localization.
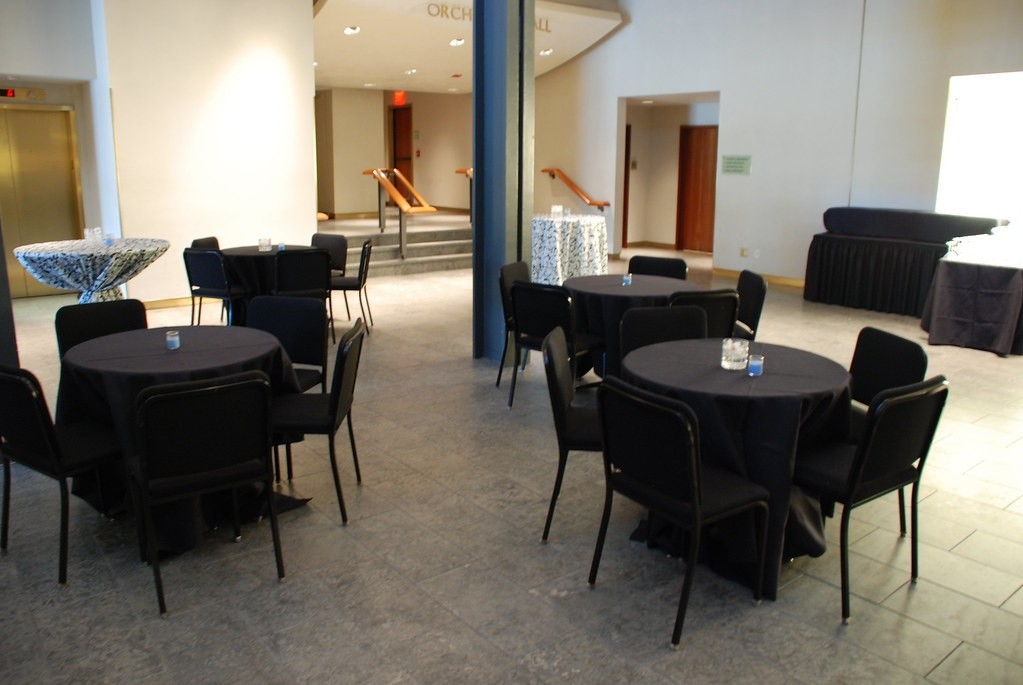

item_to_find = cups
[167,330,180,349]
[622,273,632,286]
[721,338,748,370]
[85,227,103,249]
[257,237,272,252]
[278,244,285,251]
[551,205,562,218]
[749,355,764,376]
[564,208,571,217]
[104,234,112,247]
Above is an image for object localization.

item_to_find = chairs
[670,292,739,339]
[0,362,121,583]
[494,264,530,386]
[588,376,770,650]
[628,255,687,279]
[182,248,220,324]
[54,300,146,358]
[246,291,329,478]
[326,240,374,332]
[849,325,928,404]
[191,236,218,252]
[272,247,336,344]
[618,303,709,346]
[507,281,599,410]
[738,270,767,342]
[312,232,351,321]
[277,320,362,524]
[790,375,949,623]
[542,326,603,540]
[125,369,286,618]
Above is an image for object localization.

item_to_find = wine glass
[944,236,968,261]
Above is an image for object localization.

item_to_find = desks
[531,215,608,286]
[621,339,853,605]
[13,238,169,302]
[563,275,700,372]
[51,325,314,565]
[919,249,1023,356]
[220,245,320,324]
[803,233,947,318]
